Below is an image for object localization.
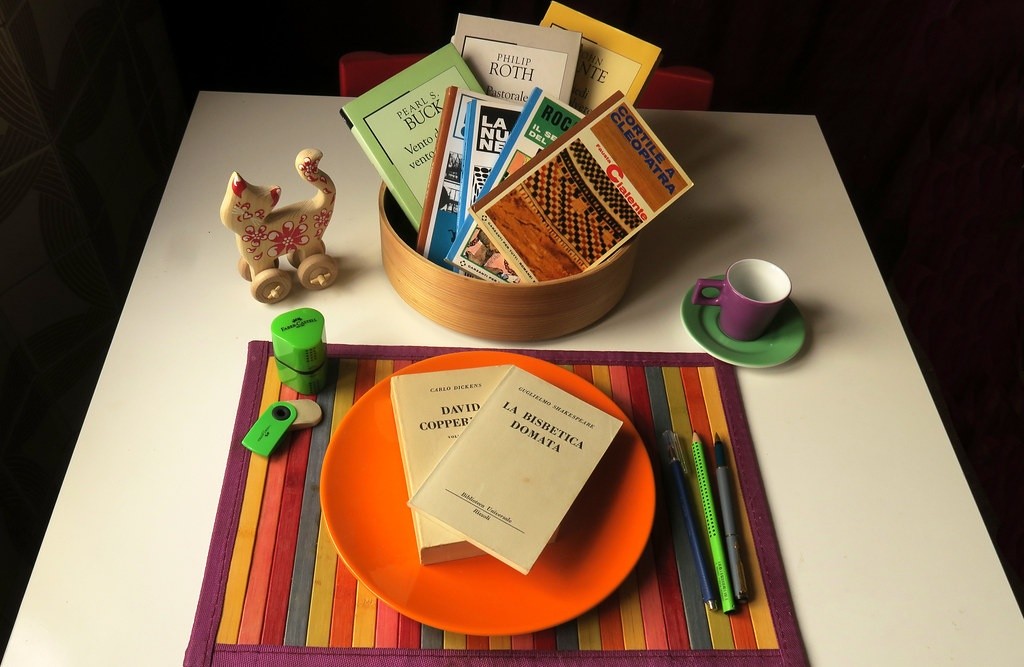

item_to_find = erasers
[241,399,322,460]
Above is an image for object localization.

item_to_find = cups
[692,258,792,341]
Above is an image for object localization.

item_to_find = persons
[853,1,1024,580]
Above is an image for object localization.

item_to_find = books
[390,366,562,564]
[404,364,623,575]
[339,0,694,286]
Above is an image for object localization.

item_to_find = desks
[0,90,1024,667]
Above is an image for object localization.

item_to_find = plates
[680,273,807,369]
[320,351,657,636]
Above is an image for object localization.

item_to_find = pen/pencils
[690,428,736,614]
[714,431,755,603]
[661,428,718,611]
